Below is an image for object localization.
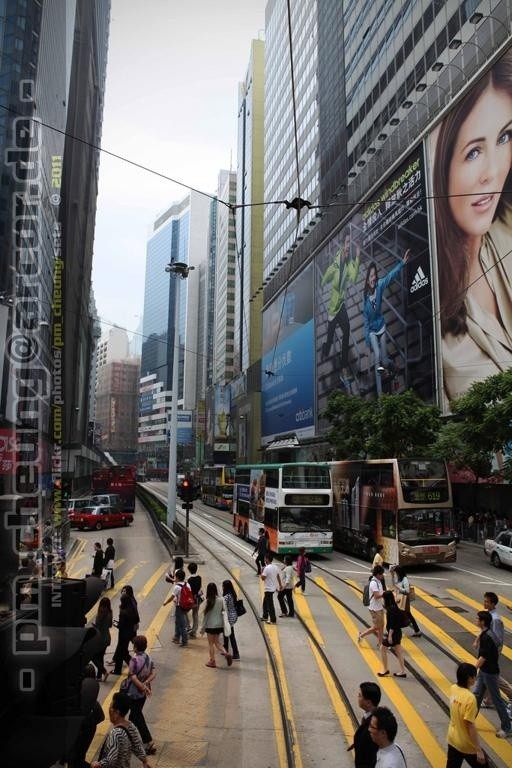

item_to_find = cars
[52,490,134,532]
[484,528,511,573]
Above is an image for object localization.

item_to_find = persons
[319,226,362,365]
[250,479,258,521]
[361,248,411,412]
[431,46,512,412]
[258,474,266,506]
[19,507,512,768]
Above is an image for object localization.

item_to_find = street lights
[239,413,248,465]
[161,254,198,530]
[194,433,202,470]
[377,366,394,396]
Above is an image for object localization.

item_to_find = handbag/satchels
[221,597,232,637]
[235,599,247,617]
[385,585,407,611]
[408,582,416,602]
[120,678,130,692]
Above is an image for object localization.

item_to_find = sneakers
[496,727,512,739]
[410,632,424,637]
[292,587,312,596]
[505,705,512,720]
[358,632,362,647]
[171,629,196,648]
[206,659,216,668]
[480,702,495,709]
[145,742,157,754]
[220,652,240,666]
[96,659,122,683]
[259,613,295,625]
[376,642,392,651]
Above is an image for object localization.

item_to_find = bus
[200,451,463,573]
[89,462,138,511]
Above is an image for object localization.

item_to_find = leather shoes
[377,670,390,676]
[394,672,407,678]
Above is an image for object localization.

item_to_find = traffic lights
[2,606,102,767]
[19,575,110,727]
[177,484,183,496]
[181,477,194,501]
[192,482,202,501]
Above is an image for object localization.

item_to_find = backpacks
[362,579,379,606]
[176,581,195,610]
